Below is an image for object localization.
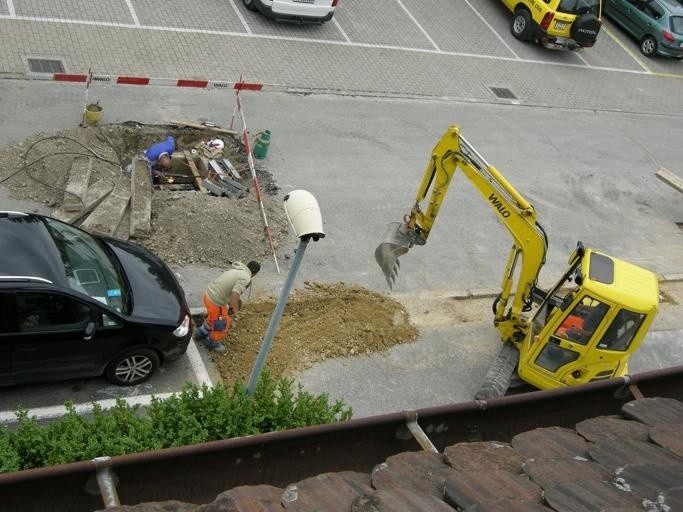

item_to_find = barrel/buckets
[85,104,103,125]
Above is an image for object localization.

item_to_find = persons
[554,301,624,346]
[191,260,261,354]
[143,135,175,177]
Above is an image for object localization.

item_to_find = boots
[194,320,212,340]
[206,336,227,354]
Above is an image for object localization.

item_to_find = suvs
[501,1,602,51]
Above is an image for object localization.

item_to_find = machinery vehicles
[375,123,661,400]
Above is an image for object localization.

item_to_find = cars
[246,0,338,25]
[601,1,683,59]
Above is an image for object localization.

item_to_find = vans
[0,211,193,386]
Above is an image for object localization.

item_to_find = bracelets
[577,328,581,338]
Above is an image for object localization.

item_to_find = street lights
[245,190,324,397]
